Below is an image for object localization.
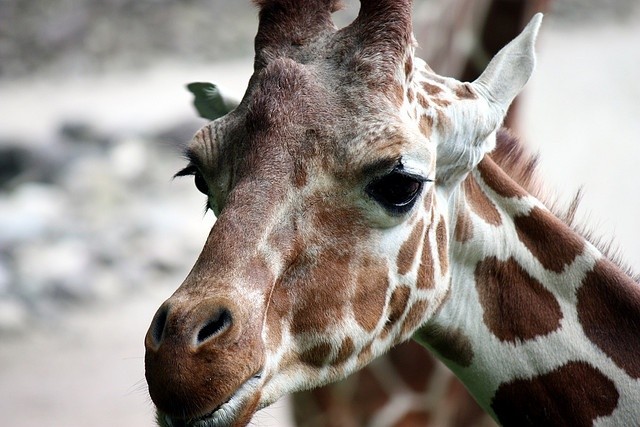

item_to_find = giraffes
[129,0,639,427]
[294,0,550,427]
